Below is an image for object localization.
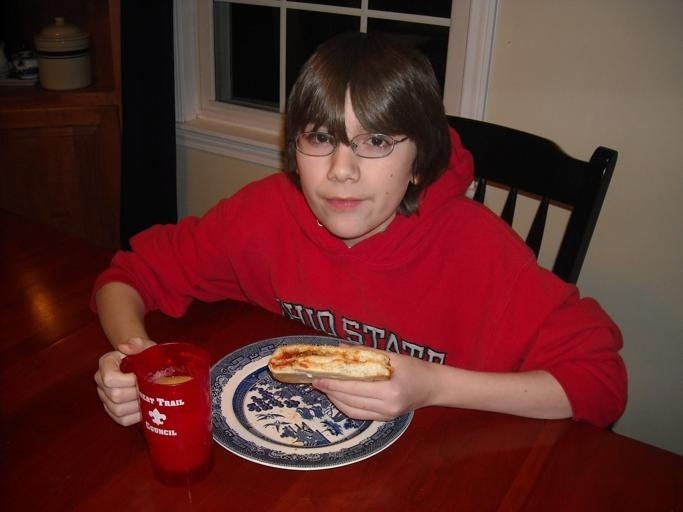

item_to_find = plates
[209,334,417,472]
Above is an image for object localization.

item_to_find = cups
[120,342,213,487]
[12,50,40,82]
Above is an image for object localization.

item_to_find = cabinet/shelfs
[1,101,126,254]
[0,0,122,102]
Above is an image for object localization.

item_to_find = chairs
[444,116,618,290]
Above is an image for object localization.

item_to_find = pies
[268,344,396,384]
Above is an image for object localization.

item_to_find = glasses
[293,125,412,160]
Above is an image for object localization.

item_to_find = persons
[93,39,628,427]
